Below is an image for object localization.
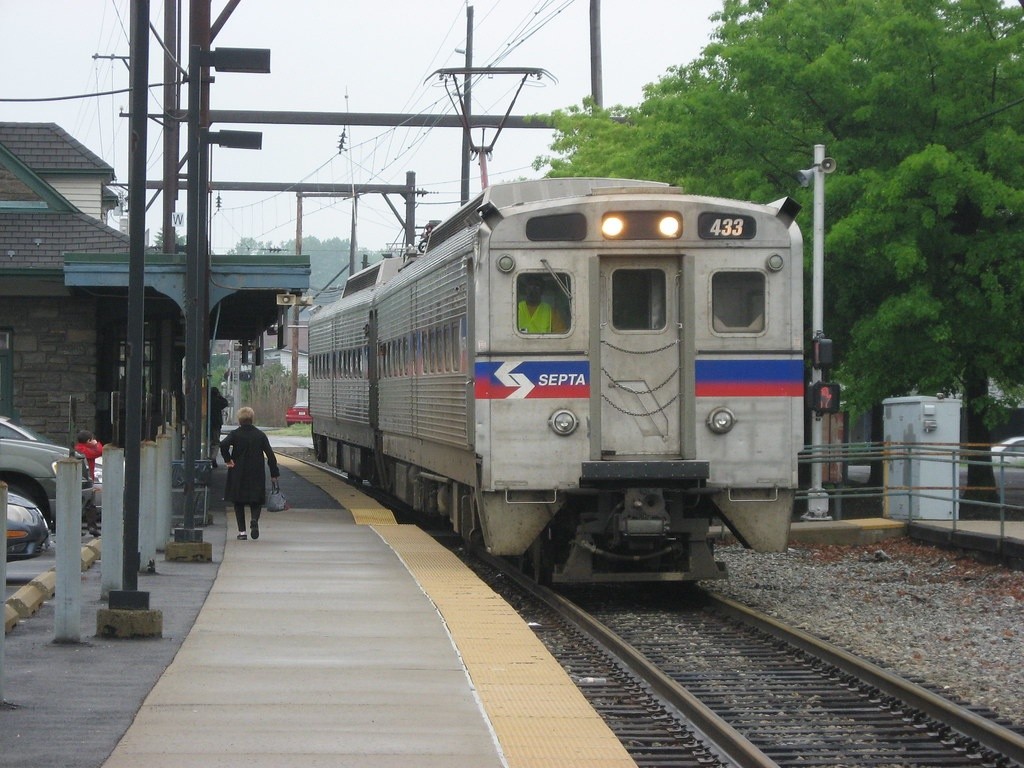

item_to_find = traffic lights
[815,380,842,415]
[814,336,835,371]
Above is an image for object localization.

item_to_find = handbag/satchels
[267,480,286,512]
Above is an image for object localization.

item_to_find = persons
[75,430,102,536]
[518,276,567,333]
[211,387,228,469]
[220,407,281,540]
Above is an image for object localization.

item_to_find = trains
[306,177,805,595]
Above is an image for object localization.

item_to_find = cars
[0,415,102,519]
[286,401,311,428]
[6,492,52,564]
[989,437,1024,468]
[0,437,94,534]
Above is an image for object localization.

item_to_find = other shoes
[237,532,247,541]
[251,520,259,539]
[212,460,217,468]
[89,527,101,536]
